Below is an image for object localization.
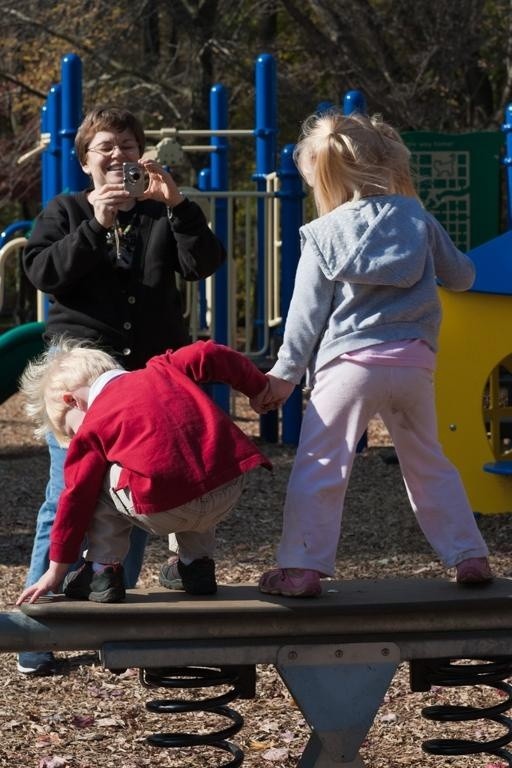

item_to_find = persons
[11,102,228,675]
[263,112,496,599]
[15,339,282,607]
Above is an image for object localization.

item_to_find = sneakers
[63,564,126,603]
[12,650,57,678]
[158,557,217,592]
[260,567,321,597]
[458,556,491,583]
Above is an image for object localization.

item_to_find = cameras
[122,162,143,199]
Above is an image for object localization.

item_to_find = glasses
[87,140,138,157]
